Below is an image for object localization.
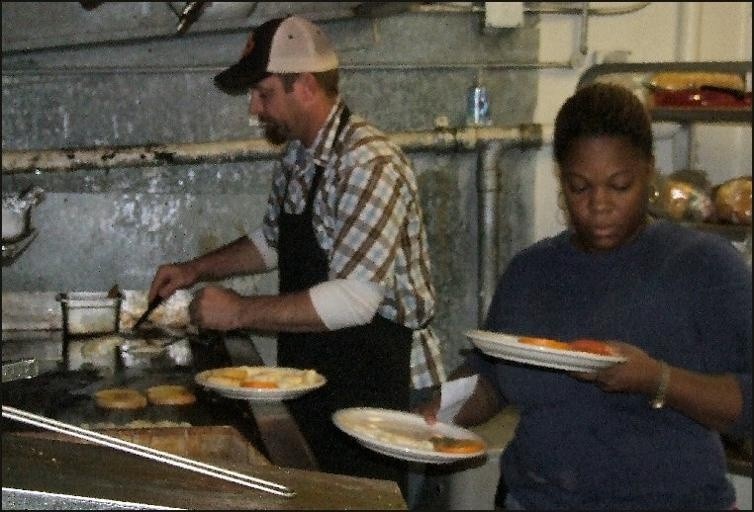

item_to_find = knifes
[133,294,165,333]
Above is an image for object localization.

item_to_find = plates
[196,366,326,399]
[463,329,627,375]
[334,405,487,466]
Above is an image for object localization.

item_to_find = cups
[466,84,495,129]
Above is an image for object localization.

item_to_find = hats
[214,15,339,96]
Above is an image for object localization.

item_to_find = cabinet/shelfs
[574,58,754,239]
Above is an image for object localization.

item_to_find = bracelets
[646,358,672,411]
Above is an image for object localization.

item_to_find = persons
[145,12,445,512]
[411,79,753,512]
